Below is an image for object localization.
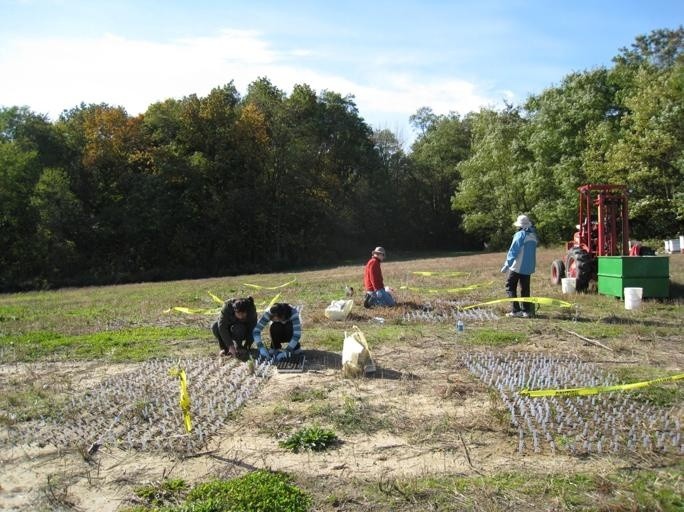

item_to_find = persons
[362,247,395,307]
[250,301,303,362]
[209,294,256,359]
[500,213,538,319]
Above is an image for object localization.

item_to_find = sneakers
[363,293,375,308]
[505,310,532,318]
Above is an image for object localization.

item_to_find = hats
[371,247,385,255]
[512,214,532,229]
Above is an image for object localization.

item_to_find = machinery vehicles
[551,180,657,292]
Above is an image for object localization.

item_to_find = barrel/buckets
[561,278,576,293]
[624,287,643,309]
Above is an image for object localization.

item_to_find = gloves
[276,352,284,360]
[259,346,268,357]
[500,265,509,273]
[375,290,382,299]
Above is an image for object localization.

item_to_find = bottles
[457,318,464,335]
